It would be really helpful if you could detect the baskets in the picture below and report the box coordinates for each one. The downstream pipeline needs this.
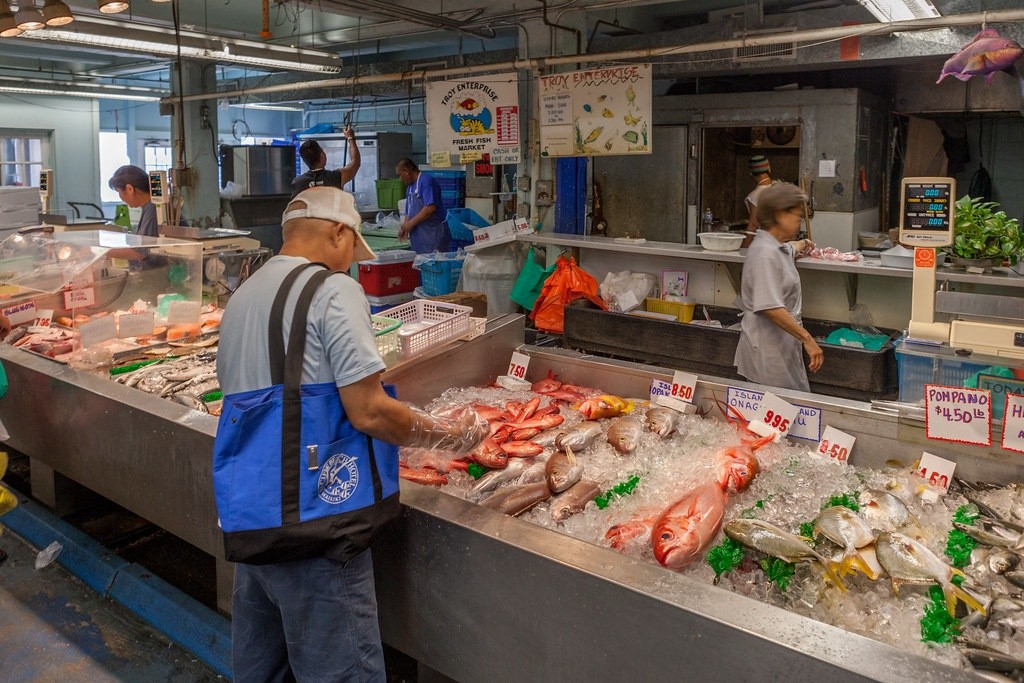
[371,314,403,357]
[646,297,696,323]
[377,298,473,357]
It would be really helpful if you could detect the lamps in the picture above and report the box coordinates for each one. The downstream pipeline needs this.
[43,0,73,26]
[97,0,130,14]
[0,0,24,38]
[19,19,343,76]
[13,0,45,31]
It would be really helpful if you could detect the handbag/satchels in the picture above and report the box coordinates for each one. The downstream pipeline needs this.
[212,262,400,562]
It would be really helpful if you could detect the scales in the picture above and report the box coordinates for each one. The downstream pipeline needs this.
[148,171,261,255]
[898,176,1024,360]
[38,169,108,232]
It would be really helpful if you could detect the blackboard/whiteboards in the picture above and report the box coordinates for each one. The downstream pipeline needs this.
[538,63,652,159]
[426,72,521,163]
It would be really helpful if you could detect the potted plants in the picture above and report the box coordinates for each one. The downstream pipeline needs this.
[945,194,1024,268]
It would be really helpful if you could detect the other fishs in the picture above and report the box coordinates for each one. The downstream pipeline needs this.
[578,394,635,421]
[608,415,642,452]
[859,470,941,538]
[943,472,1024,683]
[714,433,776,495]
[5,298,224,416]
[936,28,1023,86]
[544,450,585,492]
[603,517,653,553]
[548,480,601,525]
[877,530,987,618]
[478,482,553,518]
[393,367,601,487]
[646,406,679,439]
[721,518,859,592]
[556,421,603,452]
[812,505,877,580]
[650,481,729,570]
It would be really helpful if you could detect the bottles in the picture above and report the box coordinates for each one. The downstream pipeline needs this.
[705,208,713,223]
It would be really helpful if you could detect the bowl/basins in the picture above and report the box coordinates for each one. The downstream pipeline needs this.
[697,232,747,251]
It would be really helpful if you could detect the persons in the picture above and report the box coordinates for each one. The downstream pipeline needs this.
[217,187,489,683]
[109,164,189,272]
[741,155,777,248]
[396,158,451,254]
[733,182,824,392]
[291,124,361,200]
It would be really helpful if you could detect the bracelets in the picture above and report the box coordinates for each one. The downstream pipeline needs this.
[348,137,355,142]
[804,239,808,246]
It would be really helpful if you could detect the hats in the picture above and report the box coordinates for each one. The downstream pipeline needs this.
[750,155,771,176]
[279,186,377,261]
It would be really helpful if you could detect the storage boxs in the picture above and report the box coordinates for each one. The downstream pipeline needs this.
[446,207,491,242]
[459,317,486,342]
[421,169,466,218]
[419,253,466,296]
[374,179,405,209]
[350,227,411,280]
[646,295,696,323]
[369,315,401,359]
[359,248,418,297]
[374,300,473,359]
[365,294,415,315]
[895,351,989,404]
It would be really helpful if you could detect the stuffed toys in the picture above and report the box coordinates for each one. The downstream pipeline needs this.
[935,26,1024,85]
